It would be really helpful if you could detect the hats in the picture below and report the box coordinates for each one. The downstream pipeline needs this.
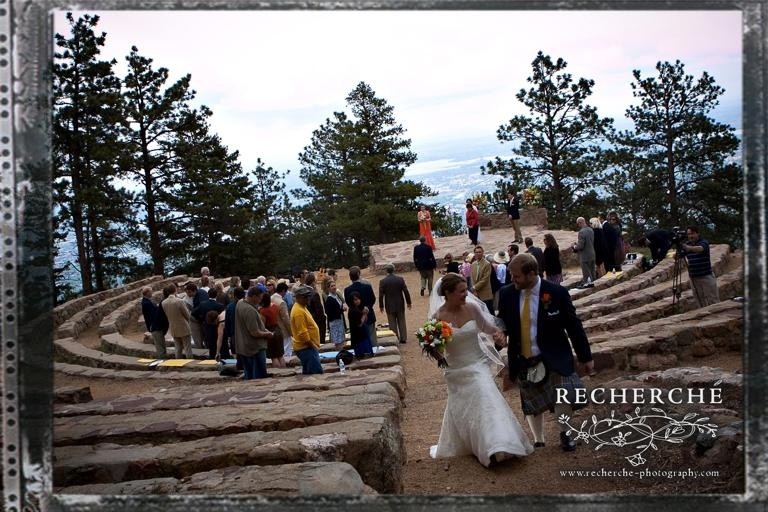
[295,288,311,295]
[493,250,511,264]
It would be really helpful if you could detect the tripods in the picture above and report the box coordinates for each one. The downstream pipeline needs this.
[672,253,703,315]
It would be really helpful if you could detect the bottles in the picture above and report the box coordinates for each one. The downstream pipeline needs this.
[339,359,345,375]
[612,268,614,274]
[586,276,590,284]
[630,255,633,264]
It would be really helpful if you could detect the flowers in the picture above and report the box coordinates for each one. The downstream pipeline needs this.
[410,316,461,370]
[519,183,544,211]
[469,192,490,212]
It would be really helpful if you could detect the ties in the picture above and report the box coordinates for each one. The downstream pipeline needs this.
[519,289,533,360]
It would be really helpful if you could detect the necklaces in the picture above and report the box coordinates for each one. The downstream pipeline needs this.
[453,315,458,322]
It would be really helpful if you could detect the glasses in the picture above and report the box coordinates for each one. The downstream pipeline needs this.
[609,218,616,221]
[265,284,273,287]
[443,256,451,259]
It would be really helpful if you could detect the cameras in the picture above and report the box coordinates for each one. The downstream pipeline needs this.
[571,242,579,254]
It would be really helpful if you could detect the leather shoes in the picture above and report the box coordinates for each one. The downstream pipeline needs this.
[559,429,577,450]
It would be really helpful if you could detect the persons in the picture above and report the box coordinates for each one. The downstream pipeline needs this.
[609,216,625,272]
[588,217,606,277]
[596,211,618,272]
[672,224,720,309]
[139,266,376,382]
[465,198,483,241]
[606,211,625,264]
[417,204,436,252]
[378,263,413,345]
[490,252,598,452]
[412,235,438,298]
[427,270,536,464]
[568,216,597,286]
[507,192,524,244]
[465,203,482,248]
[439,233,564,318]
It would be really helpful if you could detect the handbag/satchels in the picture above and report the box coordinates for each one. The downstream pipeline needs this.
[336,348,354,366]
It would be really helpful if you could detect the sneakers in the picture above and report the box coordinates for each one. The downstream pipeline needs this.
[421,288,424,296]
